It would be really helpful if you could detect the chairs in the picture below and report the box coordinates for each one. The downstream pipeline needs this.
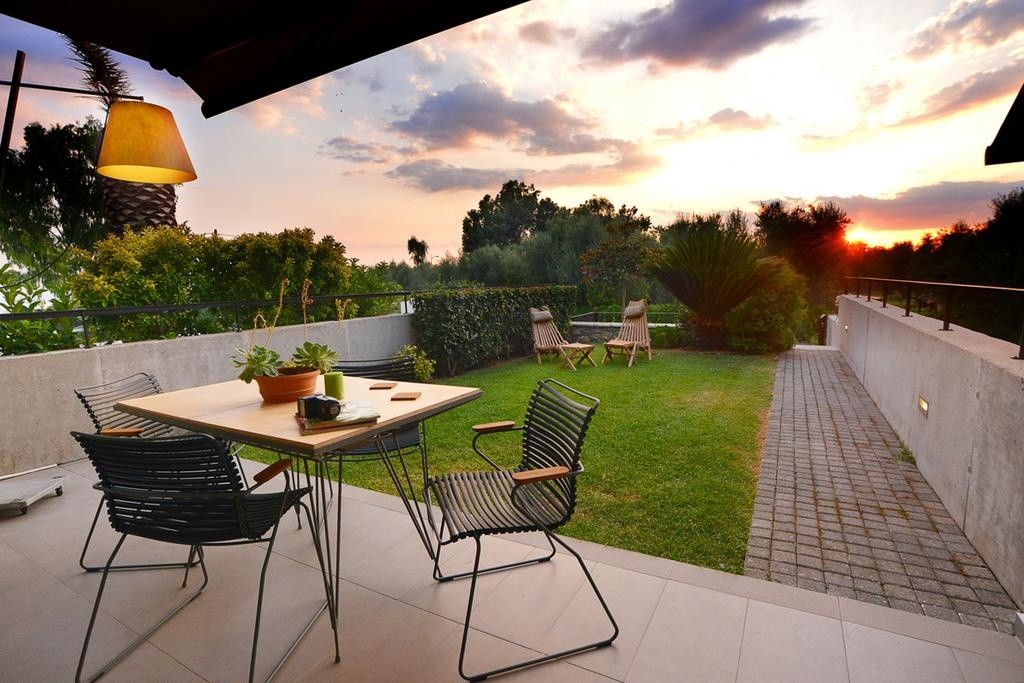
[70,431,333,683]
[529,306,597,371]
[601,299,652,368]
[74,372,251,570]
[315,354,437,533]
[422,378,619,683]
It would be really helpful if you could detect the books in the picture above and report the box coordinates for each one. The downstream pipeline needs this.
[295,399,380,435]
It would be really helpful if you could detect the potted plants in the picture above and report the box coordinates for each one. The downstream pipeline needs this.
[232,342,338,402]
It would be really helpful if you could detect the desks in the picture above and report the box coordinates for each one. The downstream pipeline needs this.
[114,375,482,663]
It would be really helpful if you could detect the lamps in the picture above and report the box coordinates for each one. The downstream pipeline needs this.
[0,80,197,184]
